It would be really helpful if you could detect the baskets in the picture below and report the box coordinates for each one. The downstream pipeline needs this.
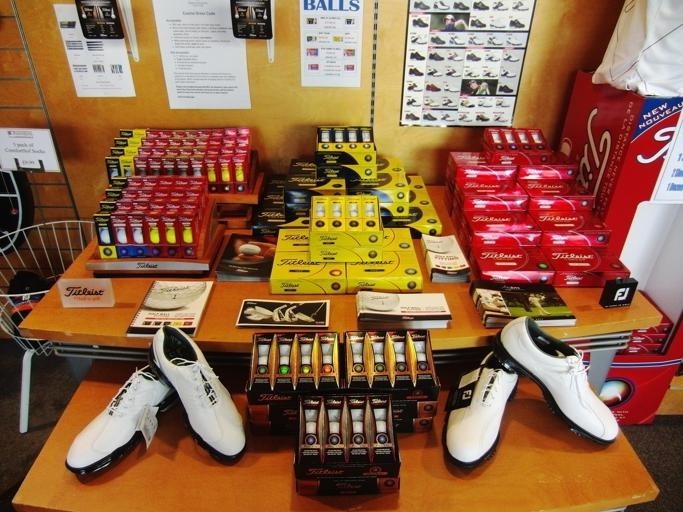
[0,220,92,356]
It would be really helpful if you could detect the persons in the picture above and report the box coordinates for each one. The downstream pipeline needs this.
[442,14,466,31]
[467,80,491,95]
[234,238,277,260]
[516,292,553,318]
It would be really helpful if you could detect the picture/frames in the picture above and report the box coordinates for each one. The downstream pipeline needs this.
[400,0,537,129]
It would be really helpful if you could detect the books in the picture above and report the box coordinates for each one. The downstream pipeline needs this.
[125,278,215,337]
[214,230,278,283]
[354,291,453,330]
[420,230,472,284]
[234,297,331,331]
[467,278,577,329]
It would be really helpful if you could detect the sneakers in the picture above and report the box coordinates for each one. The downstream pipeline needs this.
[65,364,179,476]
[406,0,529,121]
[442,351,520,471]
[492,317,619,445]
[149,325,246,464]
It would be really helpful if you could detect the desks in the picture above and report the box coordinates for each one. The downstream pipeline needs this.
[14,185,663,512]
